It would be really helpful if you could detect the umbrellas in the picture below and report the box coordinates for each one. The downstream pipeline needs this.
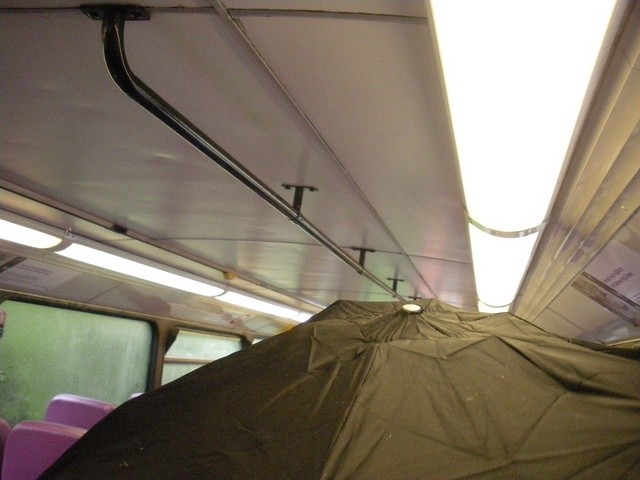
[36,299,640,480]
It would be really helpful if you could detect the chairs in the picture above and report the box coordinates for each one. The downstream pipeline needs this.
[0,418,87,480]
[45,393,117,429]
